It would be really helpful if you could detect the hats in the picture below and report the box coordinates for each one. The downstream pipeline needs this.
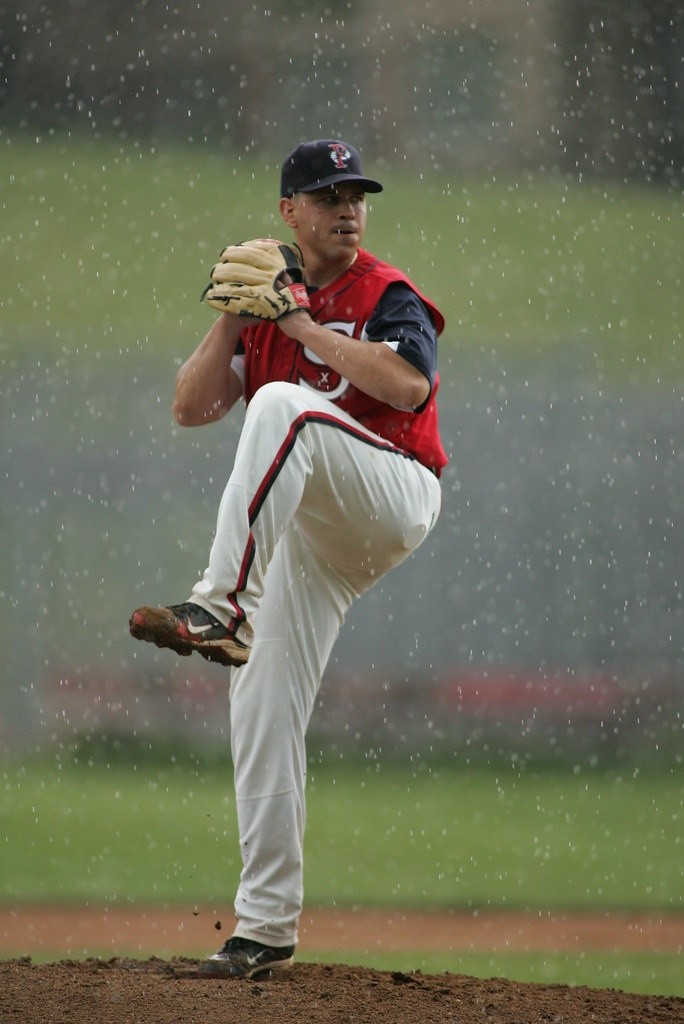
[280,139,383,198]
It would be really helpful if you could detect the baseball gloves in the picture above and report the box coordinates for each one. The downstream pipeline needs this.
[201,235,312,322]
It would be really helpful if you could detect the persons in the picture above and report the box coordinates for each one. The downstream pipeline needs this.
[126,138,448,984]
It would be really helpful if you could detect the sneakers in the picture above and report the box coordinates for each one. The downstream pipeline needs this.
[173,937,295,980]
[129,602,249,667]
